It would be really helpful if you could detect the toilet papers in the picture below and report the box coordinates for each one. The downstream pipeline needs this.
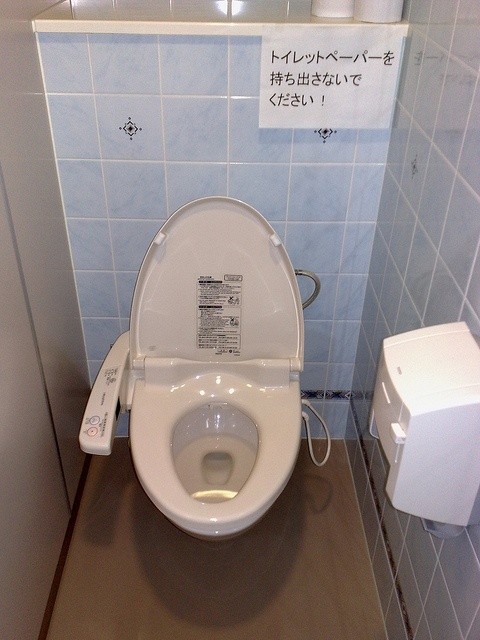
[352,1,404,24]
[311,3,354,19]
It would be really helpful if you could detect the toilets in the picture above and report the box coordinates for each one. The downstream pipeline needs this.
[77,195,305,542]
